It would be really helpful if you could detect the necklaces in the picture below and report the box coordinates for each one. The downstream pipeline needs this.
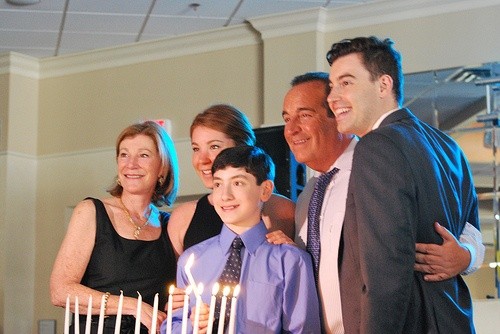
[119,198,154,240]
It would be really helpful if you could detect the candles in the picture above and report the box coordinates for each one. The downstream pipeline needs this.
[182,294,189,334]
[98,295,106,334]
[150,293,159,334]
[207,295,216,334]
[64,296,70,334]
[185,269,203,306]
[166,294,172,334]
[193,295,201,334]
[85,295,92,334]
[135,290,142,334]
[217,296,227,334]
[114,290,124,334]
[228,297,238,334]
[74,296,80,334]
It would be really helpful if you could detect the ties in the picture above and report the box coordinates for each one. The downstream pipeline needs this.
[306,167,340,290]
[212,237,245,334]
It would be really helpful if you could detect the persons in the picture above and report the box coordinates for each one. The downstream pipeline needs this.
[165,104,295,314]
[327,36,480,334]
[160,147,322,334]
[50,121,179,334]
[282,73,485,334]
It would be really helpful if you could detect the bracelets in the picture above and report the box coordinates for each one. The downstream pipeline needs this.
[104,292,110,317]
